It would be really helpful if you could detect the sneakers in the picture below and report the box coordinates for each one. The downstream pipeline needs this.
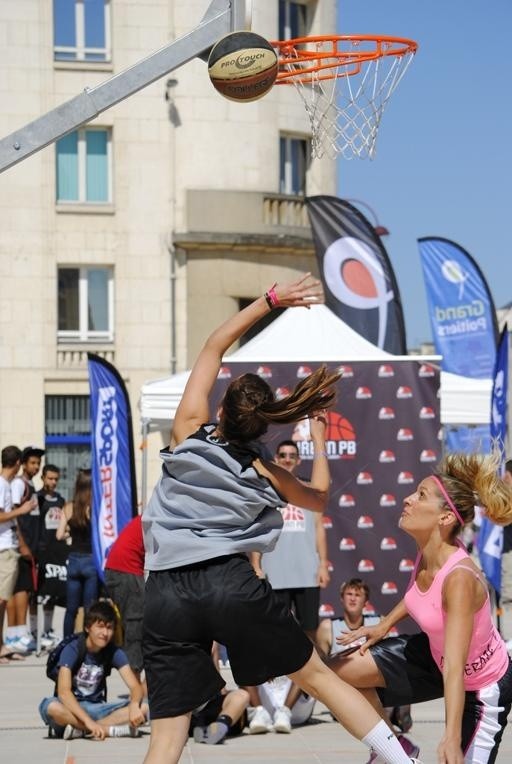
[193,721,228,745]
[114,721,139,738]
[366,735,419,764]
[272,706,291,732]
[4,632,62,652]
[63,724,85,741]
[248,706,271,735]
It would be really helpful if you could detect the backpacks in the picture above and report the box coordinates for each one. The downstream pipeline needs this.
[46,632,118,689]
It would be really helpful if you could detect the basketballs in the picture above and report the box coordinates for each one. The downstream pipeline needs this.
[292,411,355,441]
[208,30,279,103]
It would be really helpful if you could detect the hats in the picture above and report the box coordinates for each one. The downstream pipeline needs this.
[23,446,46,460]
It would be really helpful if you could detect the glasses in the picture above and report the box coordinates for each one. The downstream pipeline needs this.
[278,453,298,458]
